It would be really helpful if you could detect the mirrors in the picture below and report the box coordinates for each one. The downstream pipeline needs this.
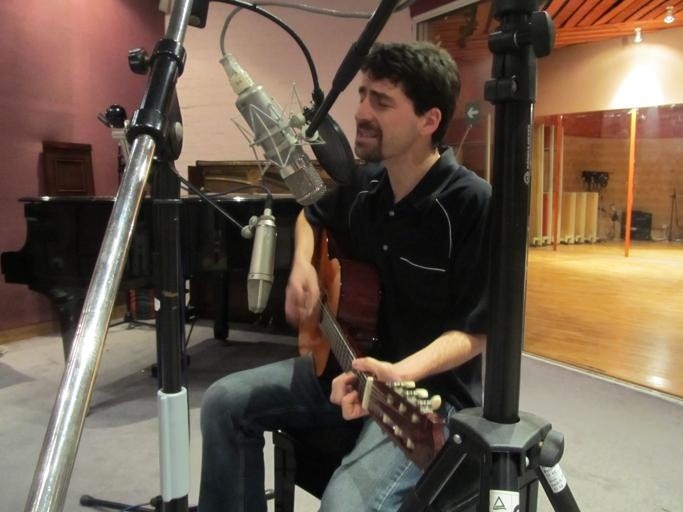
[413,1,683,404]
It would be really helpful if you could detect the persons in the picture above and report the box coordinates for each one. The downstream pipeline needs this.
[199,40,492,512]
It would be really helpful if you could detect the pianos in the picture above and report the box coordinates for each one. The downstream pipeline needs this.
[0,195,303,365]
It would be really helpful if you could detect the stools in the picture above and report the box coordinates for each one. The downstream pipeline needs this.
[273,435,483,512]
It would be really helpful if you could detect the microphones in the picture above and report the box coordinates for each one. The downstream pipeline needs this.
[244,216,278,313]
[235,84,327,206]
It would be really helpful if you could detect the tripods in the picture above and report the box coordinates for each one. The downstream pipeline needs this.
[399,2,579,510]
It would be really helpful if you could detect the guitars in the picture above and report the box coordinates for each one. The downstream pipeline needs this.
[297,225,445,469]
